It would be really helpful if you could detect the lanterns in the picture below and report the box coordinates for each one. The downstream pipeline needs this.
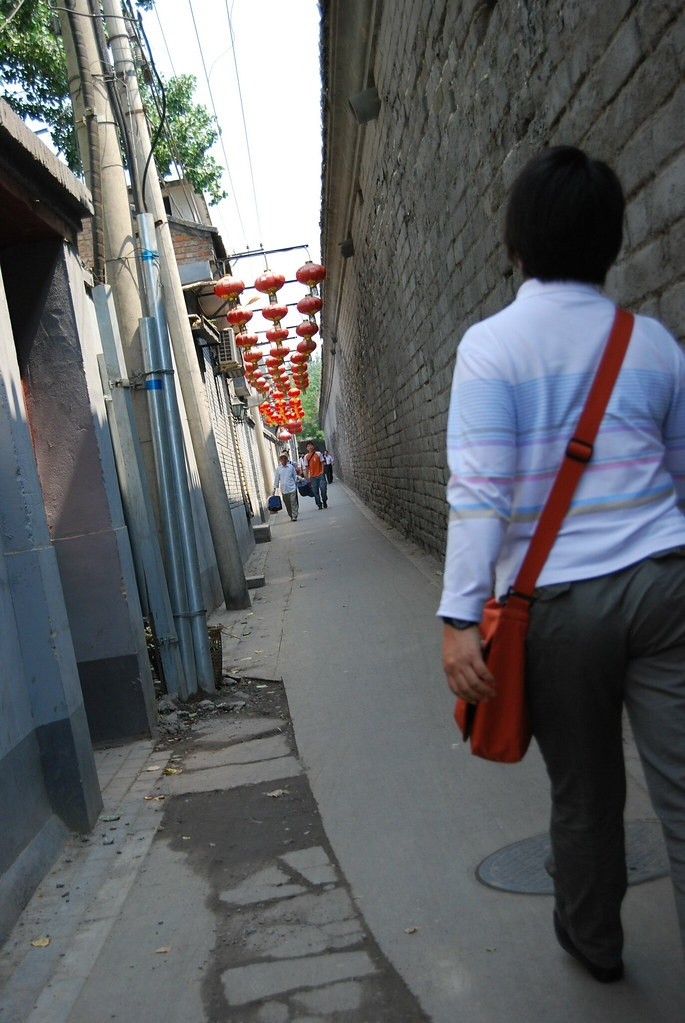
[227,303,253,332]
[270,345,290,360]
[245,352,310,435]
[266,326,289,344]
[214,273,244,301]
[297,293,322,318]
[296,261,326,288]
[279,430,292,442]
[288,388,300,398]
[235,330,258,350]
[262,300,288,325]
[297,339,317,356]
[296,319,318,339]
[243,350,262,365]
[255,269,285,296]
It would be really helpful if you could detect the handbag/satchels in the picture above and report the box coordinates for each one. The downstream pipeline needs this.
[453,597,534,764]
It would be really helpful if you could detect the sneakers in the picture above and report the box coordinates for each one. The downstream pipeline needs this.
[291,517,297,521]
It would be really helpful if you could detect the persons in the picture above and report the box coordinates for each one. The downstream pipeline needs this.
[432,146,685,983]
[278,450,299,508]
[272,452,298,521]
[304,440,328,510]
[298,450,336,484]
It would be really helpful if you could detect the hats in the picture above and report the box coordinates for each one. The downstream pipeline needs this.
[280,453,288,458]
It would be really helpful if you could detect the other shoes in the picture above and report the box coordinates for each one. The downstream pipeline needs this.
[318,502,323,510]
[323,502,327,509]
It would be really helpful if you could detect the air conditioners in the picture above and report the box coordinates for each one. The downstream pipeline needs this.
[217,327,239,369]
[233,376,252,398]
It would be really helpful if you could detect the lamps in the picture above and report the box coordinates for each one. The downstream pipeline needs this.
[230,395,245,423]
[236,406,249,424]
